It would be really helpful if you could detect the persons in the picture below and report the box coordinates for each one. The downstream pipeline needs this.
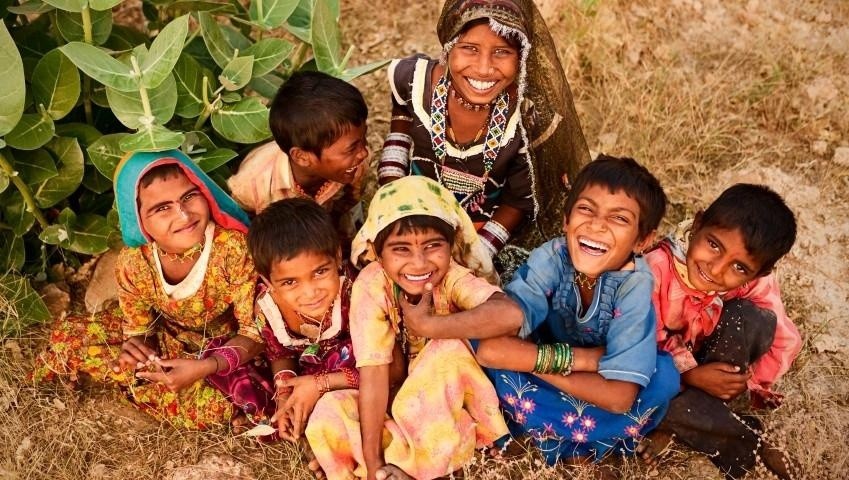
[200,197,362,448]
[222,70,374,263]
[376,0,552,257]
[643,182,808,480]
[470,153,686,480]
[33,147,272,428]
[305,174,525,480]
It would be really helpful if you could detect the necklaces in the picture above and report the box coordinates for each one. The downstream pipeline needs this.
[448,81,498,112]
[152,236,207,264]
[294,302,335,358]
[575,269,597,290]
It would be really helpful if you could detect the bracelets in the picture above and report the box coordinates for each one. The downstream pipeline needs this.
[273,368,298,401]
[314,367,330,396]
[210,346,240,377]
[209,355,219,375]
[532,342,575,379]
